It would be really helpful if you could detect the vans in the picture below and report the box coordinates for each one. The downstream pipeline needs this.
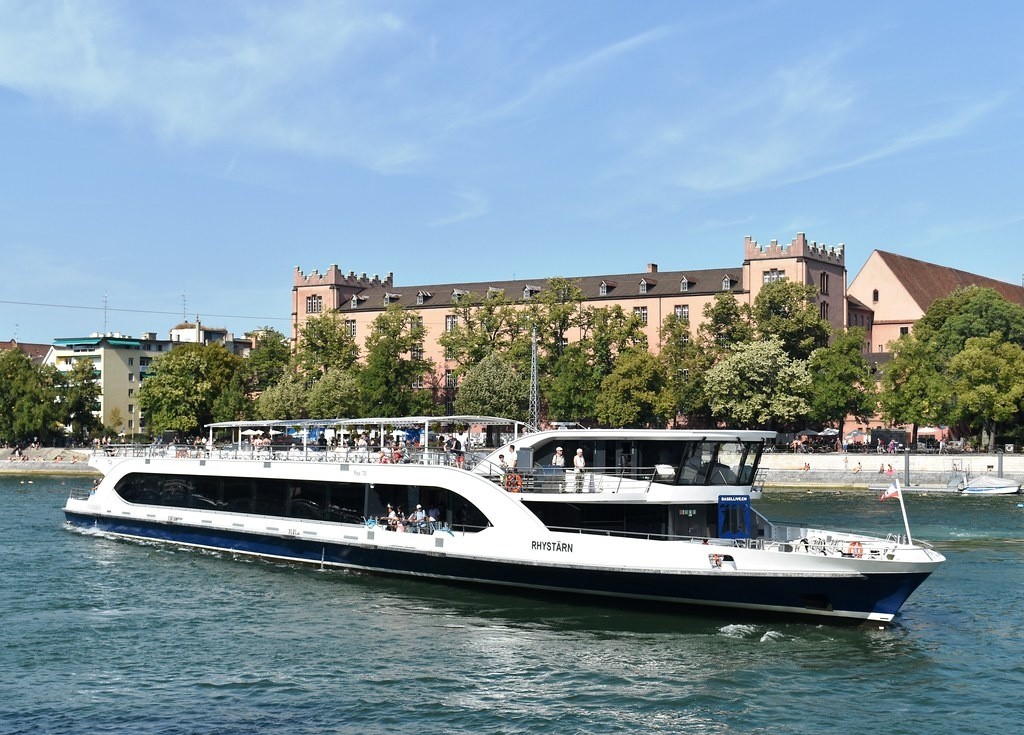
[947,441,964,453]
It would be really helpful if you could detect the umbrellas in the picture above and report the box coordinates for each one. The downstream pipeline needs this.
[849,430,862,435]
[799,428,819,435]
[817,428,840,435]
[918,427,938,448]
[241,428,309,438]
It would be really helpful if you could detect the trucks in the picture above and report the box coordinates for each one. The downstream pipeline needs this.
[722,442,746,453]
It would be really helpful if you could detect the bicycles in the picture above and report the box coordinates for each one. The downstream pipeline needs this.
[988,445,1003,456]
[817,444,833,453]
[798,444,814,454]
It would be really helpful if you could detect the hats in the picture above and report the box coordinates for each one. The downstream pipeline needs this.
[577,448,582,453]
[417,504,421,509]
[556,447,563,450]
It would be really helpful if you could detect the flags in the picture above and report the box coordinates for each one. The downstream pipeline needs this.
[879,480,900,503]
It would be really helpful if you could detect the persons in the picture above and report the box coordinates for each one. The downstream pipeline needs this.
[498,454,508,486]
[789,435,898,454]
[844,456,848,470]
[853,462,862,474]
[206,438,213,457]
[254,427,469,464]
[878,463,884,473]
[802,463,810,471]
[508,445,518,474]
[887,464,892,472]
[166,442,176,458]
[573,448,586,493]
[522,427,528,437]
[381,504,440,534]
[552,447,566,492]
[539,422,551,432]
[102,436,114,456]
[194,437,203,457]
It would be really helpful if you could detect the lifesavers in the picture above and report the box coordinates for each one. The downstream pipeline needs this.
[380,453,385,462]
[177,450,190,458]
[853,467,859,473]
[506,473,522,491]
[848,542,863,558]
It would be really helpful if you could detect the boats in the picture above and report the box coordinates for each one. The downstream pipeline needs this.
[957,473,1020,496]
[62,417,947,628]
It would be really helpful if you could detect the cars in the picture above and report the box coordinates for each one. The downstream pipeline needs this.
[750,444,771,453]
